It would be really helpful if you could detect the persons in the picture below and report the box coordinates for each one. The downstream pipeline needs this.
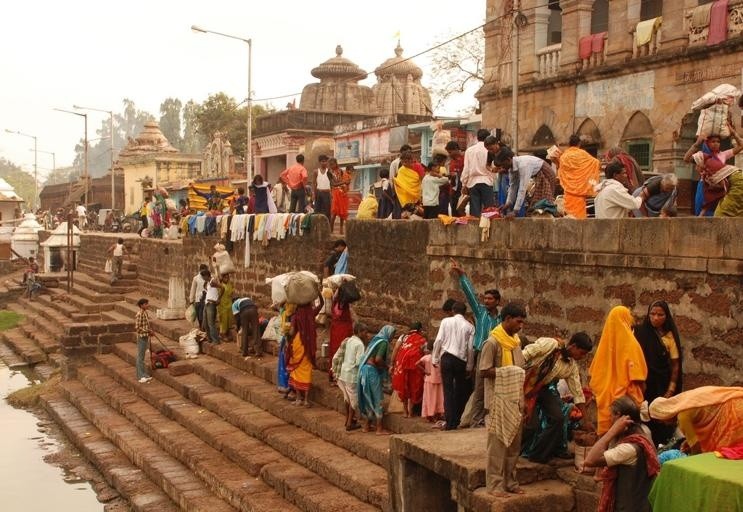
[190,239,502,435]
[24,256,42,302]
[108,238,129,283]
[135,298,154,384]
[30,154,354,239]
[355,120,743,220]
[479,300,743,512]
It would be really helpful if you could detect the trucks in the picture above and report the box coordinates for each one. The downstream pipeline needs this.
[95,209,124,231]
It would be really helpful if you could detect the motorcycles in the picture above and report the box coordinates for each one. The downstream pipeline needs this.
[106,215,131,233]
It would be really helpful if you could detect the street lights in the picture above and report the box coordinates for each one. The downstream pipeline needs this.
[72,105,116,208]
[4,128,41,211]
[25,146,56,188]
[189,24,252,199]
[50,106,89,207]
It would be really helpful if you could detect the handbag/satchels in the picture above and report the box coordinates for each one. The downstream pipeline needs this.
[694,95,730,141]
[148,333,176,371]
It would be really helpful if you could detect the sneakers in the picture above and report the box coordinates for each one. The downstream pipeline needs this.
[136,376,152,384]
[276,385,292,395]
[344,418,361,431]
[290,399,313,408]
